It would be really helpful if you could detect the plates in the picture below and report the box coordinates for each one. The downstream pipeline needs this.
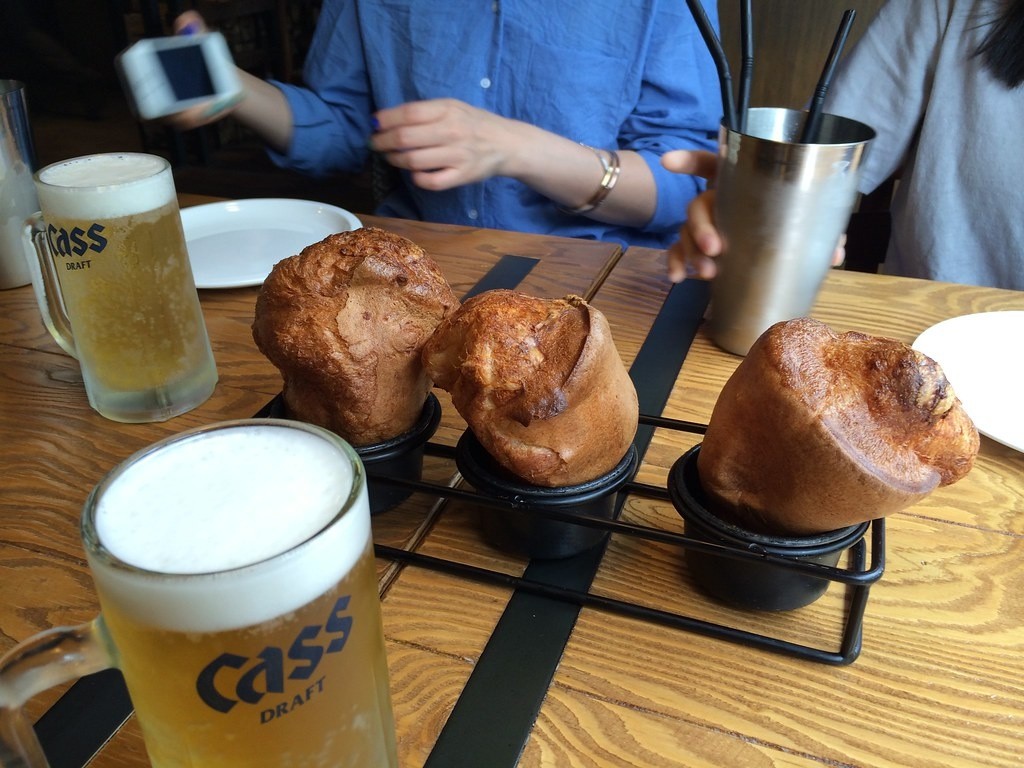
[179,196,364,288]
[911,311,1024,454]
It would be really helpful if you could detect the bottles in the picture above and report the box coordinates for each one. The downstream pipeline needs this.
[0,98,45,290]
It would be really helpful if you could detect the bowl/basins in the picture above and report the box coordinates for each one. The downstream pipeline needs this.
[668,441,871,612]
[453,426,640,562]
[352,391,442,515]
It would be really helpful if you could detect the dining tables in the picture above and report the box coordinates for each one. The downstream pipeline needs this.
[1,190,1023,768]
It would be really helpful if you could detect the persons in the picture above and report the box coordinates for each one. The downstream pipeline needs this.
[660,0,1024,291]
[169,0,725,250]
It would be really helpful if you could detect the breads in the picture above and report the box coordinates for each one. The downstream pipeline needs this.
[419,288,639,487]
[698,316,979,536]
[253,228,459,449]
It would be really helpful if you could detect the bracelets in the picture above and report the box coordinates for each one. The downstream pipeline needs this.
[558,143,619,214]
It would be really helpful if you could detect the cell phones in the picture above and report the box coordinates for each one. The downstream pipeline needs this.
[124,31,237,121]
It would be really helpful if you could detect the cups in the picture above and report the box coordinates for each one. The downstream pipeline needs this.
[0,418,396,768]
[0,79,34,172]
[22,152,219,423]
[710,106,879,360]
